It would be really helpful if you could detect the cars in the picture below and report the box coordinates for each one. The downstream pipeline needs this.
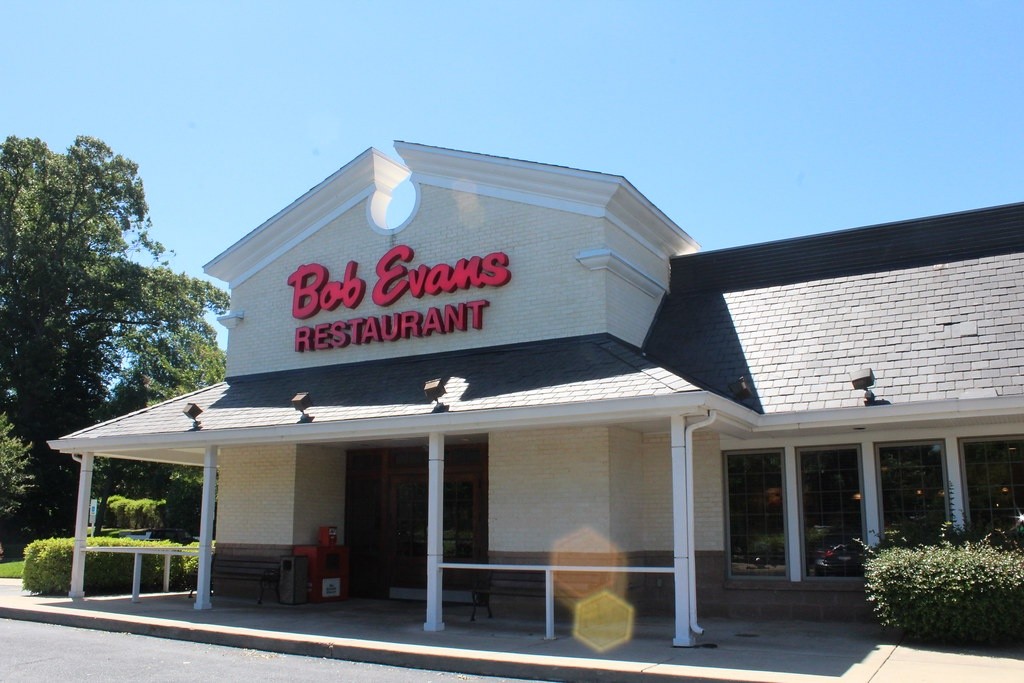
[148,528,192,544]
[810,537,865,578]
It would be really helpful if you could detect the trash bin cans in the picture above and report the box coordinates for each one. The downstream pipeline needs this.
[278,555,309,604]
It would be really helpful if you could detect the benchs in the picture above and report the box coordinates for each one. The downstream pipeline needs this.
[184,548,285,607]
[469,572,571,621]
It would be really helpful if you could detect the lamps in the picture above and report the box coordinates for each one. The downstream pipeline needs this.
[850,368,875,403]
[183,403,203,427]
[291,392,310,420]
[424,378,446,410]
